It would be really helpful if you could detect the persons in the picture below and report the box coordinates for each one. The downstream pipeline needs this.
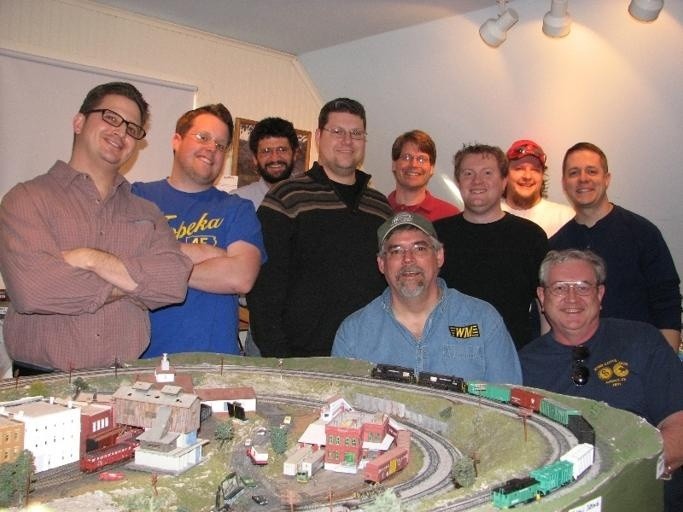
[532,141,678,351]
[499,141,576,238]
[384,128,462,226]
[1,83,187,372]
[512,249,681,472]
[223,116,299,353]
[247,100,398,356]
[124,102,268,355]
[425,143,547,349]
[327,212,523,386]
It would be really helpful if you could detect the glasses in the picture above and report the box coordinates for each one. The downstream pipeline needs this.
[183,131,231,154]
[85,108,147,141]
[255,147,291,156]
[512,146,546,162]
[383,241,433,258]
[321,127,368,140]
[542,280,597,297]
[396,153,429,164]
[569,345,590,386]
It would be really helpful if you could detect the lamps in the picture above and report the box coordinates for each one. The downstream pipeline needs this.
[475,0,664,49]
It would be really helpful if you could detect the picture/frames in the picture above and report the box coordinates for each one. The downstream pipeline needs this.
[229,117,312,190]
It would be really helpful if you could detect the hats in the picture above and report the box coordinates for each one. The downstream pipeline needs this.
[506,139,546,168]
[376,210,438,249]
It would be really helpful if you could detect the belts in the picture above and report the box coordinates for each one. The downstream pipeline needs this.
[11,361,55,379]
